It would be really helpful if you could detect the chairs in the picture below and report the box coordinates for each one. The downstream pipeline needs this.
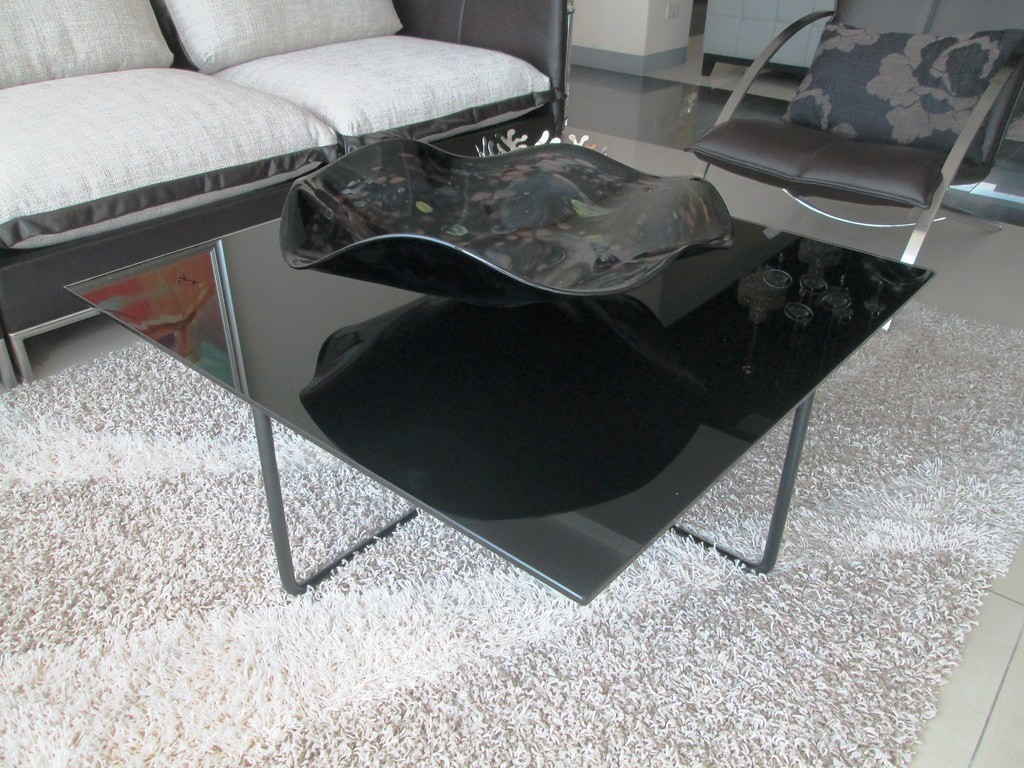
[682,0,1024,274]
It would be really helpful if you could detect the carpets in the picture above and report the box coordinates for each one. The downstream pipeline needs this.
[0,300,1024,768]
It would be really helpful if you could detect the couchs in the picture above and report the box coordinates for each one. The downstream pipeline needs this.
[702,0,836,75]
[0,0,573,391]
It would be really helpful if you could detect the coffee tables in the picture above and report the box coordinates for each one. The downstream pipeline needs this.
[63,151,935,604]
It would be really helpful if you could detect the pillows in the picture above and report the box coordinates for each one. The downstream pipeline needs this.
[789,22,1024,167]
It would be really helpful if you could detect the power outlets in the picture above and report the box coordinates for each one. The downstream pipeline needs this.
[666,2,679,18]
